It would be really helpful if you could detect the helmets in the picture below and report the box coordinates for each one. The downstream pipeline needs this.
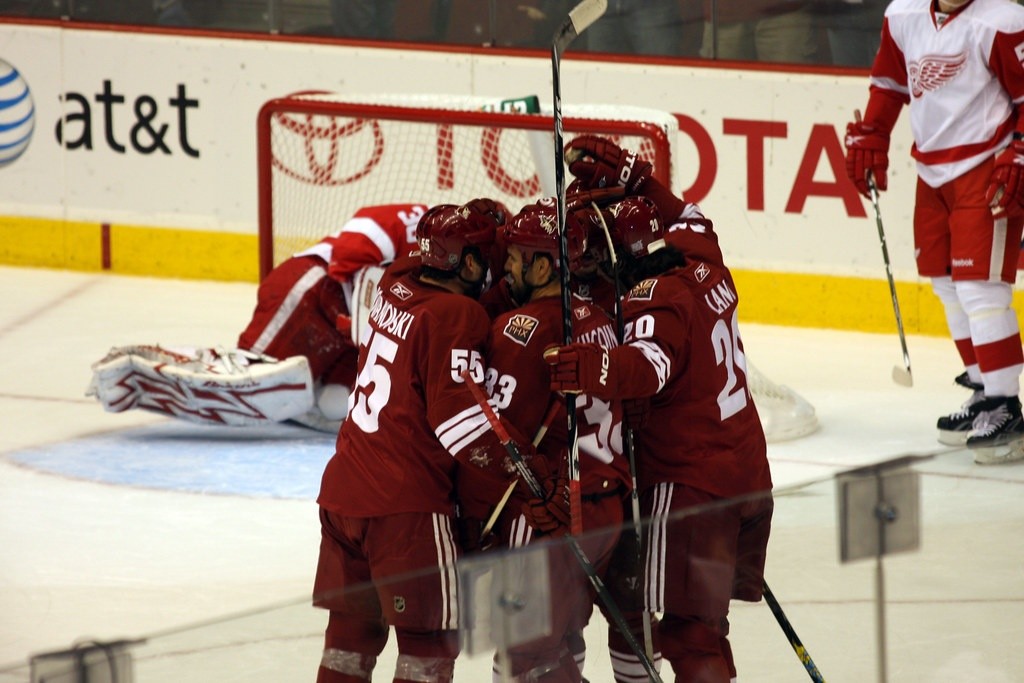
[415,194,667,290]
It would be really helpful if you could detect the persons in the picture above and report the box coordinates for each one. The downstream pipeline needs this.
[461,178,661,683]
[311,204,589,683]
[845,0,1024,466]
[461,204,632,683]
[544,135,773,683]
[0,0,890,69]
[91,204,428,425]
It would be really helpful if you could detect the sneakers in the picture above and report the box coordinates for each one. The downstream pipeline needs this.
[936,370,1024,465]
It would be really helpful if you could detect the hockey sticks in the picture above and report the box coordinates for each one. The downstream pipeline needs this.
[463,357,666,683]
[757,581,821,683]
[470,394,566,555]
[552,2,609,538]
[864,169,912,389]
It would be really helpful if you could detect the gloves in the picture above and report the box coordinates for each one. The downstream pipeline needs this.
[542,342,619,405]
[984,140,1024,221]
[844,121,891,202]
[563,135,653,194]
[517,476,571,546]
